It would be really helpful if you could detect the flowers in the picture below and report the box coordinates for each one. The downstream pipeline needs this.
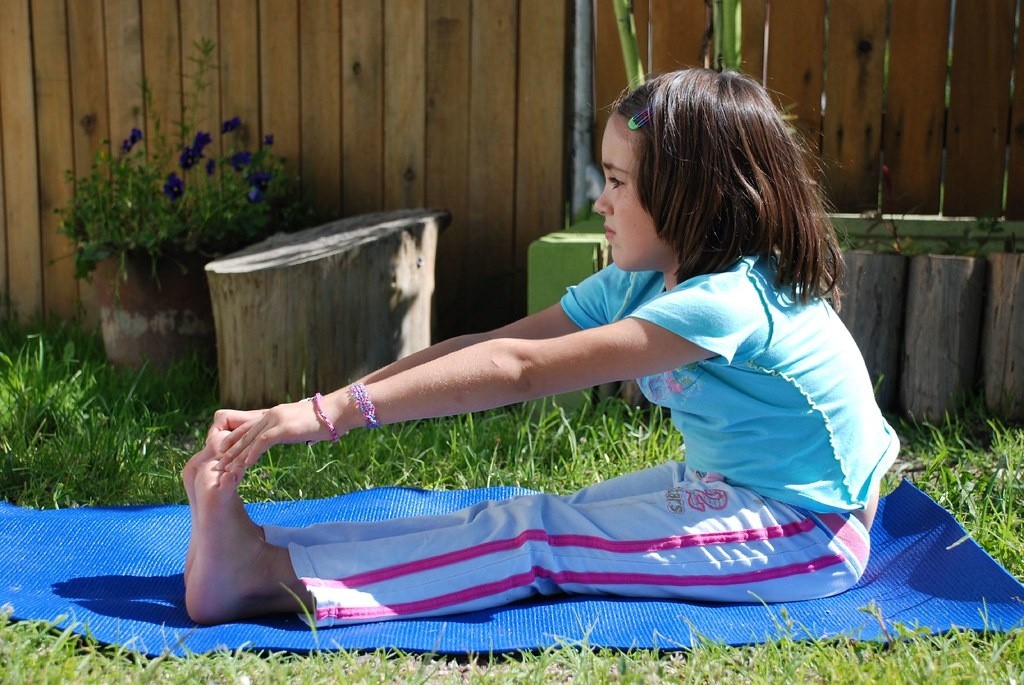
[42,33,317,302]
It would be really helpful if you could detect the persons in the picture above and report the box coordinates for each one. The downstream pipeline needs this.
[182,65,902,631]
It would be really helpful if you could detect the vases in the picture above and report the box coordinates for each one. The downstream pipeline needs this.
[89,254,217,365]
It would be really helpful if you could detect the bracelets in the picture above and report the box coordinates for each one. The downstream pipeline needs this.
[308,392,339,447]
[348,382,382,429]
